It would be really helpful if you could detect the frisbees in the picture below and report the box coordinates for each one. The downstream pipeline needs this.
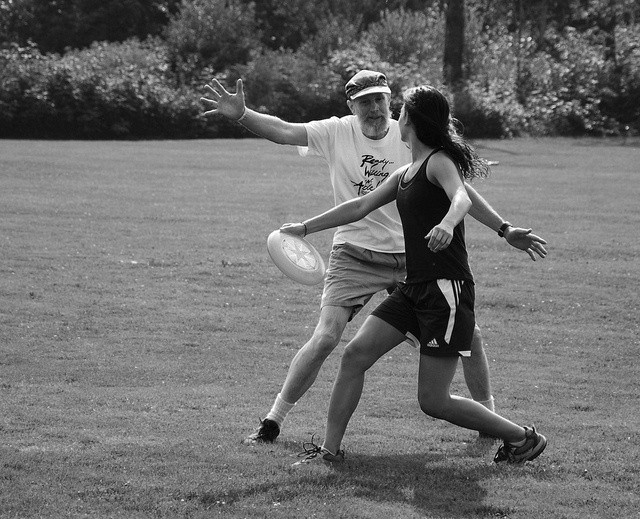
[266,229,327,285]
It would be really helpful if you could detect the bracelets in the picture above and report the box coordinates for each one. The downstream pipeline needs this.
[236,106,247,123]
[300,222,308,237]
[498,221,513,237]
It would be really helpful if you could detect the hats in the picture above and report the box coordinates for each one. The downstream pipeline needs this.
[345,70,392,99]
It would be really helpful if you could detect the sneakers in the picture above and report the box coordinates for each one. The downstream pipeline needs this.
[292,445,345,465]
[491,425,547,465]
[244,418,280,444]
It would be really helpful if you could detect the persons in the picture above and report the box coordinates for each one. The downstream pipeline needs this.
[200,69,549,446]
[280,85,548,469]
